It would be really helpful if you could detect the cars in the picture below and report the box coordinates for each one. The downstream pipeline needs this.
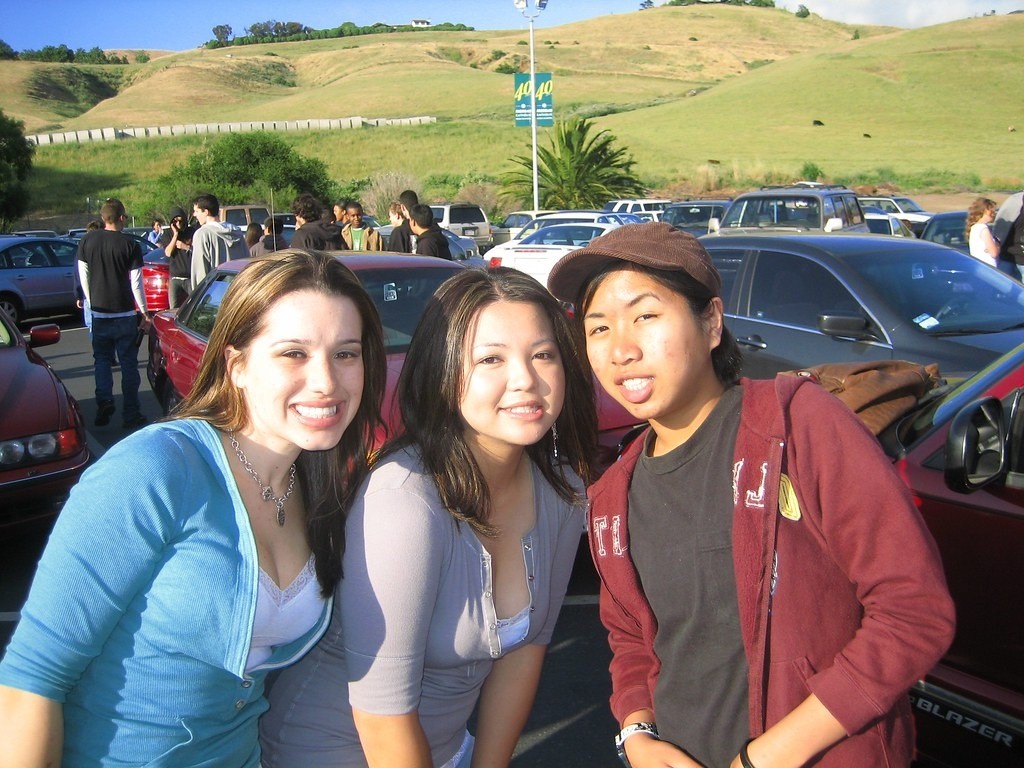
[145,248,650,598]
[691,230,1024,400]
[874,336,1024,768]
[0,304,94,544]
[1,180,1023,322]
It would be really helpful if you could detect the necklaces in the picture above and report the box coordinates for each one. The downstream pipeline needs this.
[227,430,297,527]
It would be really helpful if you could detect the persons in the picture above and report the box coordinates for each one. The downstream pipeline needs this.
[190,193,251,291]
[249,216,289,258]
[290,194,350,251]
[163,206,197,309]
[548,222,955,768]
[340,202,386,250]
[0,248,390,768]
[334,198,350,228]
[320,210,336,224]
[147,219,170,251]
[259,263,603,768]
[244,223,263,248]
[388,200,407,252]
[73,220,138,367]
[409,204,453,261]
[392,189,441,253]
[964,197,1000,269]
[77,198,152,429]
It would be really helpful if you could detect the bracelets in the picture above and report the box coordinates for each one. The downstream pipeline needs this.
[739,737,755,768]
[185,245,190,254]
[615,722,659,768]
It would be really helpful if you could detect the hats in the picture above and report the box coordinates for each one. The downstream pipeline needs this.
[549,222,722,303]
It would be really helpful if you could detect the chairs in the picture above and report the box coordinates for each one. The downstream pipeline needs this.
[766,204,787,224]
[943,231,966,245]
[29,252,49,268]
[377,269,428,336]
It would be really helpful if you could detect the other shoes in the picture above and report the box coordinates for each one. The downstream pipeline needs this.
[95,400,116,427]
[123,414,147,430]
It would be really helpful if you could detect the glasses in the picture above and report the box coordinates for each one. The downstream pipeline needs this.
[173,220,184,224]
[123,215,128,219]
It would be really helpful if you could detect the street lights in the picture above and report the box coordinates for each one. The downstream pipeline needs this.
[513,0,549,213]
